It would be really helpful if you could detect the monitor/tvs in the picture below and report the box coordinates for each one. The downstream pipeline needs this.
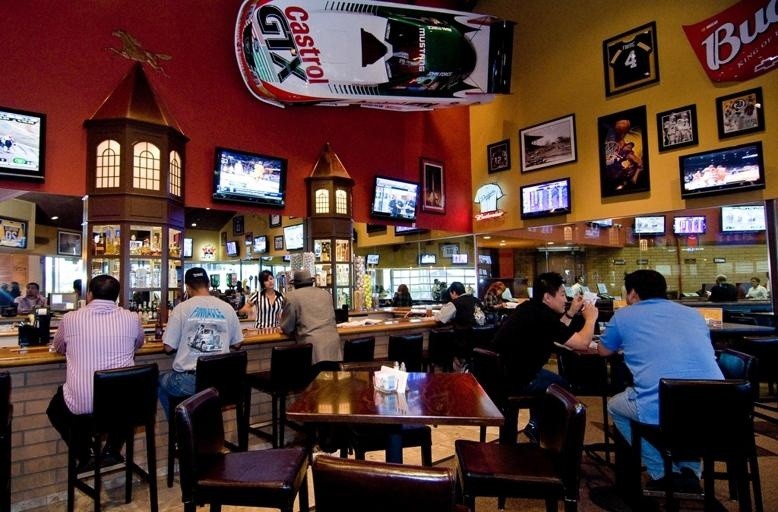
[584,203,770,239]
[173,224,309,264]
[597,282,608,296]
[369,175,421,220]
[678,139,767,200]
[210,145,289,209]
[350,224,469,268]
[0,106,48,184]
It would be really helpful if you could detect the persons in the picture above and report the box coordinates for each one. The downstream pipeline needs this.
[73,279,83,298]
[235,278,242,293]
[477,269,598,441]
[484,280,508,319]
[707,273,738,303]
[15,282,49,316]
[280,269,345,380]
[0,281,19,308]
[235,270,284,333]
[568,274,587,297]
[594,268,727,491]
[723,96,758,133]
[743,276,769,300]
[662,111,693,145]
[45,273,146,473]
[434,280,485,364]
[156,264,244,428]
[606,134,643,191]
[390,283,413,307]
[687,164,738,188]
[389,195,415,217]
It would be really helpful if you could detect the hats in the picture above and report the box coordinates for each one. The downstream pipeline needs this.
[184,267,209,284]
[288,269,317,286]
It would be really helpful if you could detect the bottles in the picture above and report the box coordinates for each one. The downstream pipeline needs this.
[313,242,350,310]
[154,317,163,339]
[91,226,181,256]
[289,252,316,282]
[168,302,173,317]
[128,300,157,319]
[113,260,177,287]
[353,255,380,311]
[392,361,409,392]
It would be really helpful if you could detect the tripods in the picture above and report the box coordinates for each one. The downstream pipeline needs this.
[519,177,572,219]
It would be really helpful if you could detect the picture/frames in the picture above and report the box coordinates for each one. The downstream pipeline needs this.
[215,209,283,256]
[601,19,663,99]
[55,229,82,258]
[418,156,448,216]
[655,103,700,154]
[486,138,512,176]
[713,85,765,140]
[518,111,580,176]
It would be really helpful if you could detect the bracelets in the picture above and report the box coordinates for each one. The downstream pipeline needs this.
[565,311,573,319]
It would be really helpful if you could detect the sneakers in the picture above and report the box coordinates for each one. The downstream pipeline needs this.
[524,420,539,445]
[75,451,112,480]
[92,451,127,472]
[646,470,685,494]
[680,466,704,494]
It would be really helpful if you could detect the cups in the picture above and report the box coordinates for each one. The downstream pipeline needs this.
[426,307,432,317]
[36,315,51,343]
[705,318,721,328]
[19,325,38,345]
[371,373,397,394]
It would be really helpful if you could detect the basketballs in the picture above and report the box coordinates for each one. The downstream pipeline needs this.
[613,119,630,136]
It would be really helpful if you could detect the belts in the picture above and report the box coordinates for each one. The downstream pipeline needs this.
[178,368,198,375]
